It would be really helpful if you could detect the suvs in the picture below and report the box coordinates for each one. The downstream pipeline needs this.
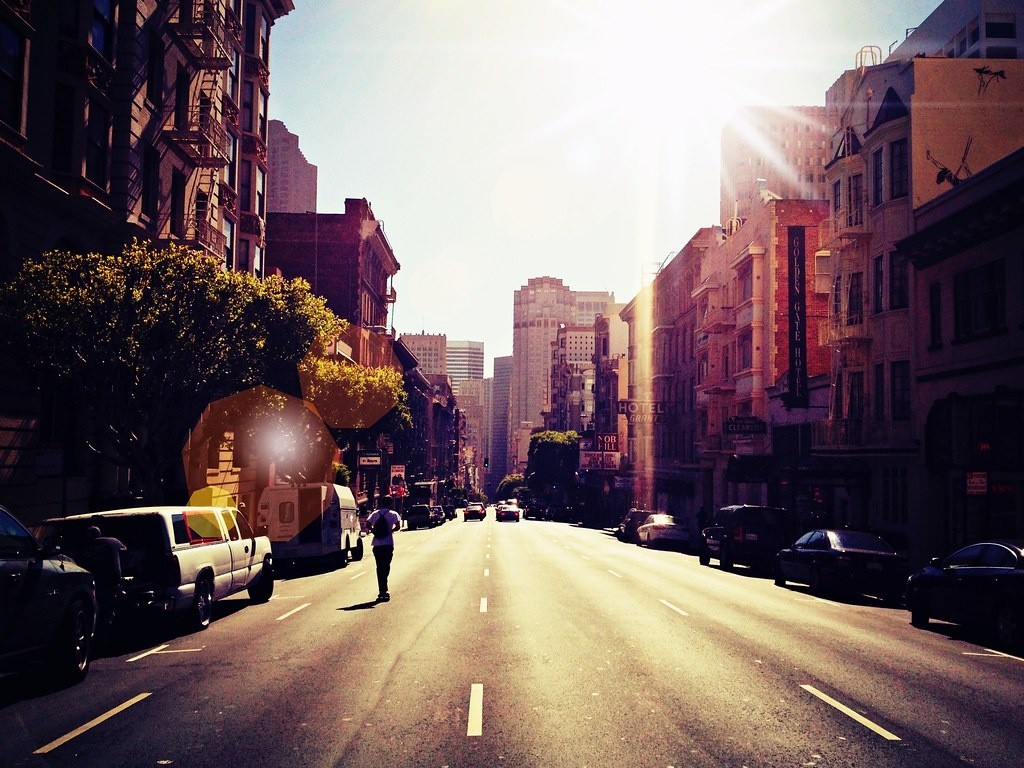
[618,508,656,542]
[701,504,788,575]
[0,504,98,690]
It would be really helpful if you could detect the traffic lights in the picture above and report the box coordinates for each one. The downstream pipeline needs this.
[483,457,490,467]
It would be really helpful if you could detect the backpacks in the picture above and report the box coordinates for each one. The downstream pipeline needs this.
[373,510,390,540]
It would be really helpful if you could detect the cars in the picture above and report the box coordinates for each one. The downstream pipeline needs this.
[905,540,1024,637]
[774,529,906,604]
[406,502,545,530]
[636,513,690,549]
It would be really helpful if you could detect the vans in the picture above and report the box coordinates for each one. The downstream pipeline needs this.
[35,504,274,638]
[257,481,364,571]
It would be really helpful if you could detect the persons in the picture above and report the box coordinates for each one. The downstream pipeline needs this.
[364,495,401,603]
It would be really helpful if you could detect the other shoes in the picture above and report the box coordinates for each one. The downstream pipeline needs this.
[378,592,390,603]
